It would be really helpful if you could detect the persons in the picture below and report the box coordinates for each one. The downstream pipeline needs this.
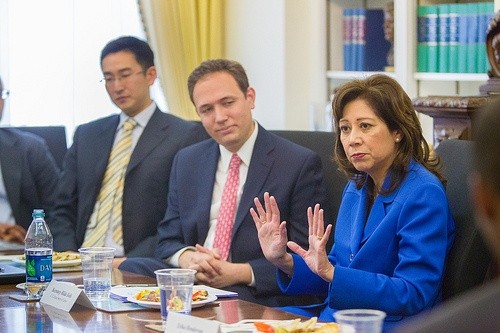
[51,35,212,275]
[398,103,500,333]
[0,77,60,250]
[250,75,456,333]
[155,59,327,307]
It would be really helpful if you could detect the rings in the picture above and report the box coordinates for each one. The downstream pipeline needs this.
[317,235,322,240]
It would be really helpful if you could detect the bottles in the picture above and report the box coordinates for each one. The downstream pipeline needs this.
[24,209,54,296]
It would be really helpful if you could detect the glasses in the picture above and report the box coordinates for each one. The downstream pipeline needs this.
[101,70,144,84]
[0,88,10,100]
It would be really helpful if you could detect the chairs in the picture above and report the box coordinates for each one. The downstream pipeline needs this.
[9,125,68,173]
[433,138,496,304]
[265,129,349,239]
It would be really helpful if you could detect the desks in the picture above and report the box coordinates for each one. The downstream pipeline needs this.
[0,266,331,333]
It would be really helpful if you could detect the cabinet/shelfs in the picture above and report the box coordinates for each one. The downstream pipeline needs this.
[317,0,500,131]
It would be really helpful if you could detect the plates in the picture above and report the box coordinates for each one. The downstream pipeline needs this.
[126,291,217,310]
[12,255,82,268]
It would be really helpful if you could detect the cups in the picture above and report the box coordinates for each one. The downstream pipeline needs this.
[153,269,197,330]
[78,247,116,302]
[333,309,387,333]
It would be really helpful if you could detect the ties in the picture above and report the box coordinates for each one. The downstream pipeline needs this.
[211,152,243,262]
[82,118,139,249]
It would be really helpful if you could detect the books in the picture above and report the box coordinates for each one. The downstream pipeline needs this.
[416,3,494,76]
[343,8,389,73]
